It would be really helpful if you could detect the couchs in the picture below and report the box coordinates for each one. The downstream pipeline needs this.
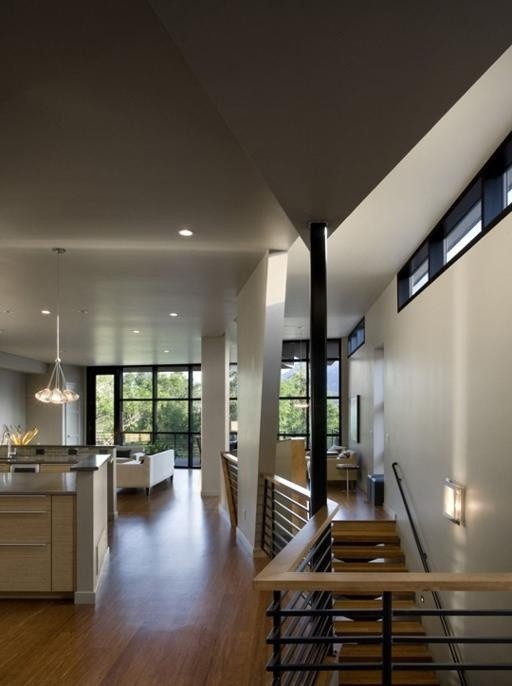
[116,449,175,498]
[306,446,356,491]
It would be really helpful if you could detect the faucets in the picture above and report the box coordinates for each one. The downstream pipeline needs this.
[1,430,17,458]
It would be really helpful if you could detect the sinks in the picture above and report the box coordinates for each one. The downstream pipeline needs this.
[0,457,18,463]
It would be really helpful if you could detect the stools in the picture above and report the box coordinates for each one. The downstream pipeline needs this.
[367,473,383,504]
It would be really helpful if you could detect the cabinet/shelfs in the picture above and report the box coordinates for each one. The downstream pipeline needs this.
[1,493,76,594]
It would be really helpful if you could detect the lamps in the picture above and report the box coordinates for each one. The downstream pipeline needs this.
[34,247,80,403]
[439,477,465,528]
[230,421,238,440]
[295,327,309,408]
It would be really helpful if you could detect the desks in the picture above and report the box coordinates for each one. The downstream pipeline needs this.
[337,464,360,494]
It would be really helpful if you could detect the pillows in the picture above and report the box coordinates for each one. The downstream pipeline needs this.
[327,448,350,459]
